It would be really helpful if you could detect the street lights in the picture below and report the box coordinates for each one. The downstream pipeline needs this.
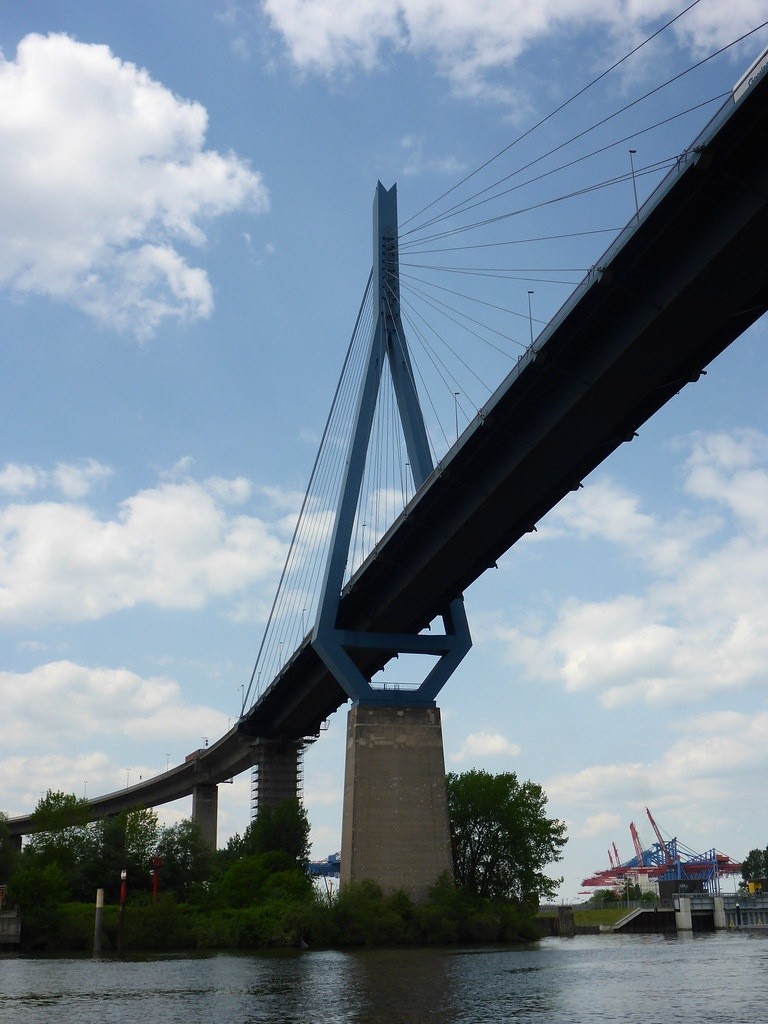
[405,462,412,507]
[629,148,638,212]
[302,607,307,641]
[258,671,262,701]
[453,390,461,443]
[165,753,171,774]
[125,768,132,788]
[279,640,283,672]
[526,289,536,346]
[83,780,89,799]
[360,522,368,563]
